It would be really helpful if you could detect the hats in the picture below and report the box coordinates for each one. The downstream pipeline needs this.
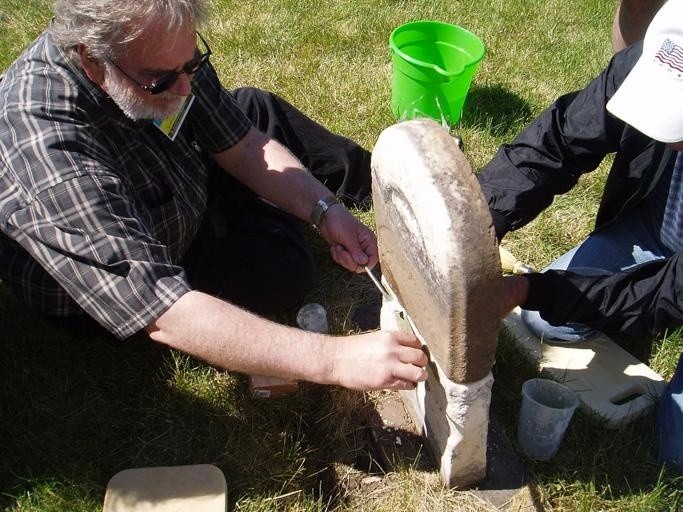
[605,1,683,145]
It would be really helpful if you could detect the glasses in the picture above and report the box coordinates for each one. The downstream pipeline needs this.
[105,29,212,95]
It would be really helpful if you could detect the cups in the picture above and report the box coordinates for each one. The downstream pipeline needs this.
[518,377,579,464]
[296,302,331,337]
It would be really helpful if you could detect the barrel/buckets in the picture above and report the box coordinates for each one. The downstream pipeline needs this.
[388,20,485,128]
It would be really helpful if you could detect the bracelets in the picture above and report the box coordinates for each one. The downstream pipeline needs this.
[310,194,342,236]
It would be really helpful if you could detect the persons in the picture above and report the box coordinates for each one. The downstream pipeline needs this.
[1,2,430,393]
[474,2,683,473]
